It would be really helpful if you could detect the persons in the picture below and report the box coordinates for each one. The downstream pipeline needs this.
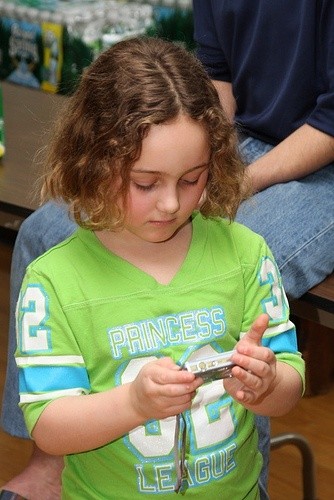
[0,0,333,500]
[0,0,334,500]
[12,31,307,499]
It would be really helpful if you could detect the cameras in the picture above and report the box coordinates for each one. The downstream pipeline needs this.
[182,350,248,383]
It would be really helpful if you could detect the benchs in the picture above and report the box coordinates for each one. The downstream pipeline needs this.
[0,79,334,315]
